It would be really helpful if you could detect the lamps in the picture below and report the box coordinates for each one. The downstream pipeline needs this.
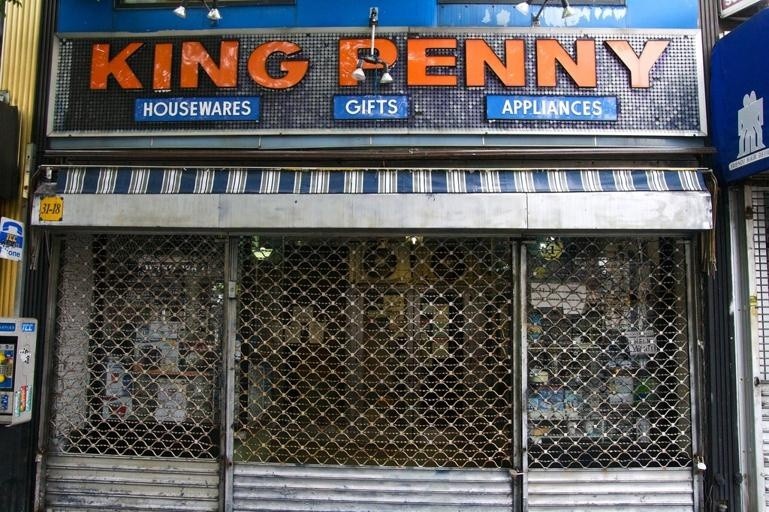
[514,0,575,26]
[350,8,394,85]
[173,1,222,25]
